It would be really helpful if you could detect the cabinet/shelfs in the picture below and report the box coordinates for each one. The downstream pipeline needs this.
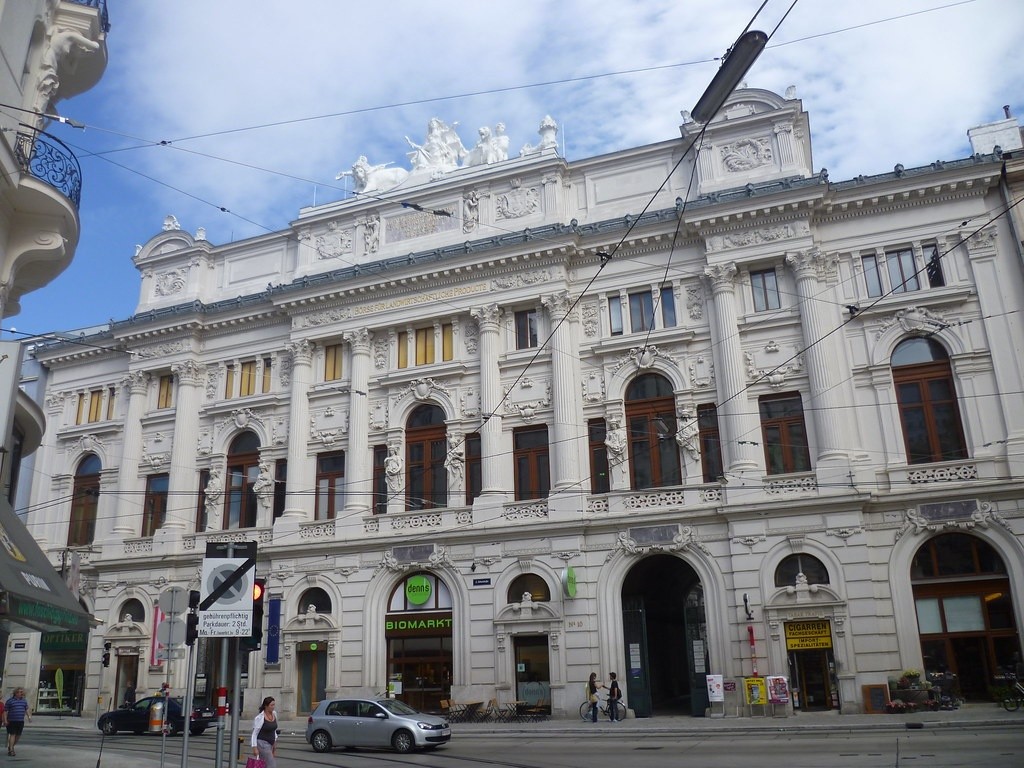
[36,688,72,712]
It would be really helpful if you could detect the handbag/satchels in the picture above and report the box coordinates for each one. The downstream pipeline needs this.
[246,754,267,768]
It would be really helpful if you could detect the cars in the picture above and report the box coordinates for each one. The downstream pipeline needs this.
[304,697,452,755]
[97,696,217,738]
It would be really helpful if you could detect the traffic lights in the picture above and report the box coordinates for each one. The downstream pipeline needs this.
[186,613,199,646]
[101,652,111,667]
[240,577,267,651]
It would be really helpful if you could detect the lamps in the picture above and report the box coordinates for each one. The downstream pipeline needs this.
[691,30,768,124]
[651,418,671,434]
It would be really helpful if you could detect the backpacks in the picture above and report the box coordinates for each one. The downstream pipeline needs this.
[617,687,622,700]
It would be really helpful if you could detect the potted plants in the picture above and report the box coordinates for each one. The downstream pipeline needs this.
[888,675,898,689]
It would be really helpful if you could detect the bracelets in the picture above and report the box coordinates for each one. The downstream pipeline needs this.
[28,716,31,719]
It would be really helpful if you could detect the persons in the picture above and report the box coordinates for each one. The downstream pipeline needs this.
[675,409,700,463]
[202,468,223,516]
[250,697,278,768]
[252,462,272,509]
[602,672,621,721]
[383,444,403,495]
[604,417,628,483]
[584,672,603,723]
[122,680,136,709]
[443,436,466,492]
[334,118,510,197]
[0,686,32,757]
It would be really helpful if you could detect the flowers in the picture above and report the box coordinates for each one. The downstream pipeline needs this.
[898,677,911,687]
[922,698,942,707]
[886,698,919,709]
[904,669,920,678]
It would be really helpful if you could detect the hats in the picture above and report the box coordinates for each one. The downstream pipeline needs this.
[610,672,616,679]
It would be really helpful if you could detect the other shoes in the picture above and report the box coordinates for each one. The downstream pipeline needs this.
[8,750,16,756]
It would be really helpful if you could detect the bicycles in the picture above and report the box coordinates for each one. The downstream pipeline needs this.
[579,694,626,722]
[1001,670,1024,712]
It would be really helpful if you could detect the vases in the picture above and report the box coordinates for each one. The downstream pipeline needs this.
[911,677,920,685]
[925,705,939,711]
[901,686,910,689]
[889,707,915,713]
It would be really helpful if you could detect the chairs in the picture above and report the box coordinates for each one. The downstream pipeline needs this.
[440,698,464,723]
[525,698,551,724]
[476,698,508,724]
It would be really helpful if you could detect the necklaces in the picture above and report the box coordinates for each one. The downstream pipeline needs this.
[264,712,273,722]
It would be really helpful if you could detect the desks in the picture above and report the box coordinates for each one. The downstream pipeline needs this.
[503,701,528,723]
[455,701,484,723]
